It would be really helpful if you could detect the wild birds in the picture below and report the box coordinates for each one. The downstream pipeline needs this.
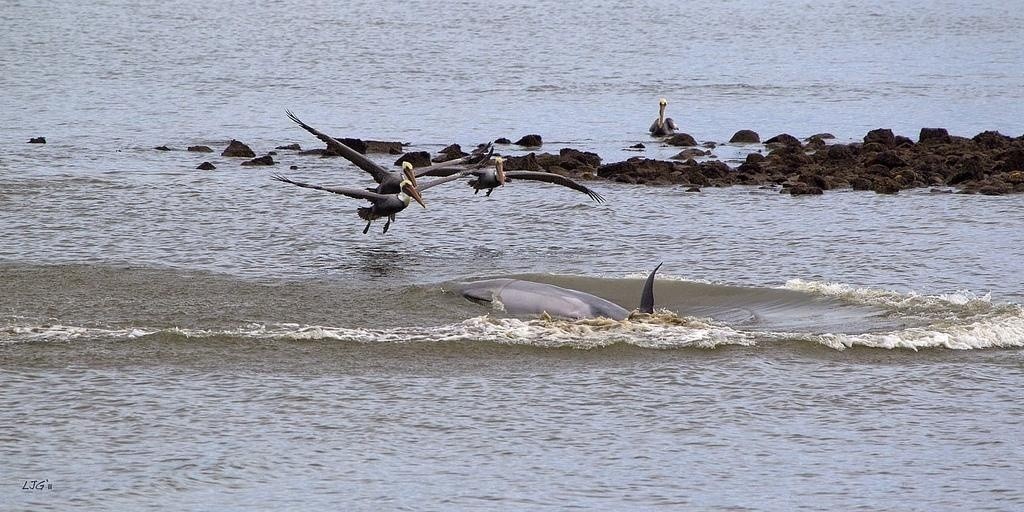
[267,145,495,234]
[649,97,682,137]
[282,106,493,194]
[384,154,609,207]
